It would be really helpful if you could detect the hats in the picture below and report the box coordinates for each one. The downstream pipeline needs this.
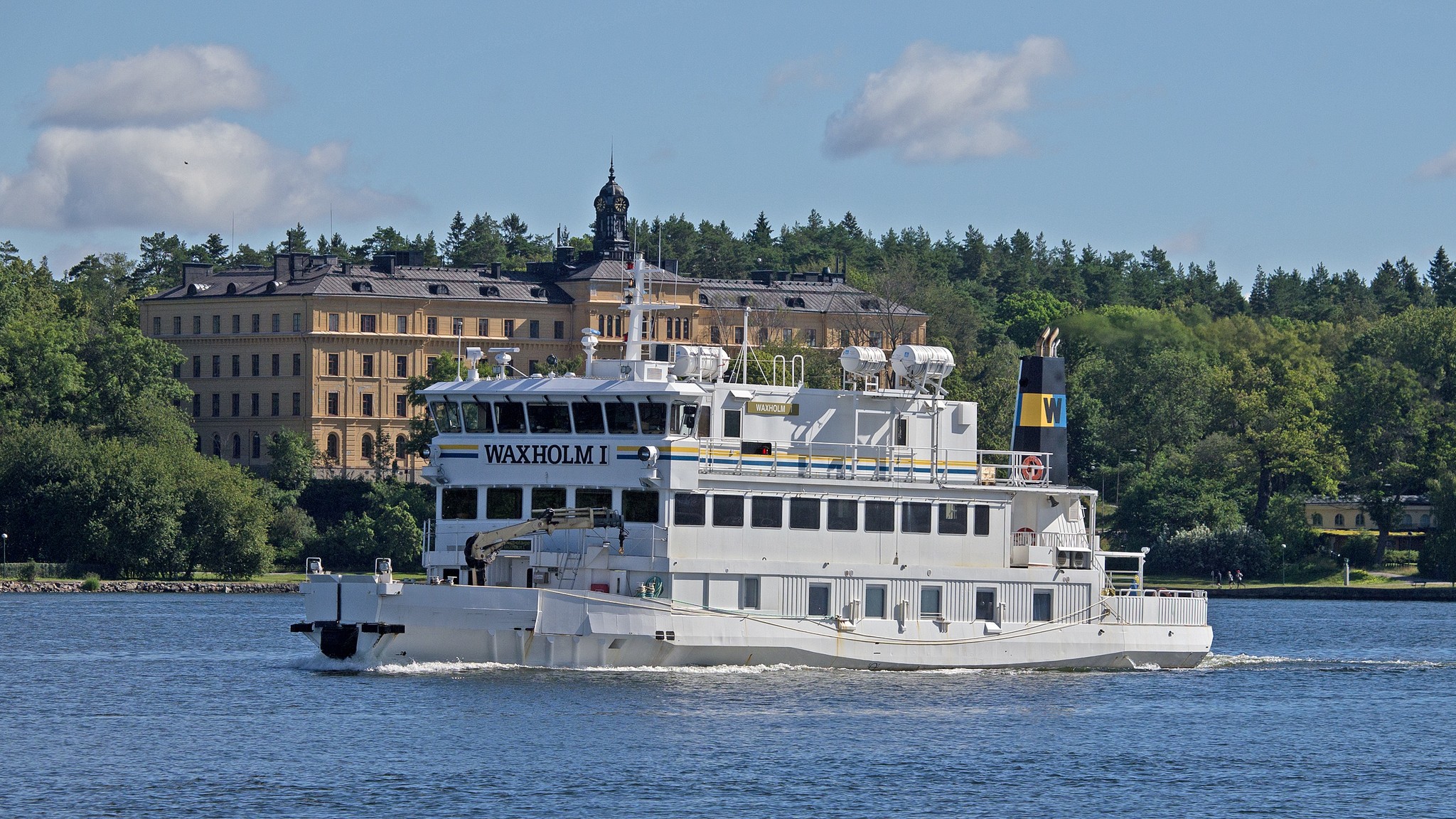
[1236,570,1240,572]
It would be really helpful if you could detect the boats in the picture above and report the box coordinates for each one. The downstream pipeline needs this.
[286,215,1210,676]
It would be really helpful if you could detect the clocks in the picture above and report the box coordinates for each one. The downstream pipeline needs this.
[595,197,605,212]
[615,197,626,212]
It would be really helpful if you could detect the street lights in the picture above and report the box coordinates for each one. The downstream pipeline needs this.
[1,534,7,579]
[1281,544,1286,586]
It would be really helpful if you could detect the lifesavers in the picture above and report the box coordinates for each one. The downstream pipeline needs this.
[1013,527,1036,546]
[1021,456,1043,480]
[1152,587,1172,597]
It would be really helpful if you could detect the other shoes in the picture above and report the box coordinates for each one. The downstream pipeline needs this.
[1233,582,1235,585]
[1230,587,1232,589]
[1218,586,1222,589]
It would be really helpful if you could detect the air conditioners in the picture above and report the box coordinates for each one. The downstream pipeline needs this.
[1057,550,1070,568]
[1070,552,1087,568]
